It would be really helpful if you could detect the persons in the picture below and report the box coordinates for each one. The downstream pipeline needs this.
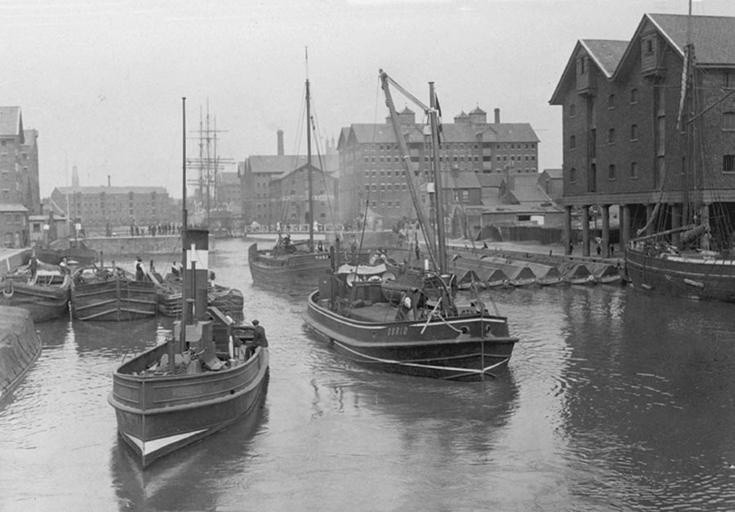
[172,260,183,276]
[279,221,291,231]
[370,248,394,268]
[136,256,146,280]
[400,291,412,314]
[315,240,325,259]
[130,222,182,235]
[392,215,420,242]
[210,272,216,288]
[349,235,359,252]
[244,320,269,361]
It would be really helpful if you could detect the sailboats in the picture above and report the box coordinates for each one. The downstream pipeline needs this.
[186,96,236,240]
[311,362,518,511]
[247,46,330,283]
[623,0,735,304]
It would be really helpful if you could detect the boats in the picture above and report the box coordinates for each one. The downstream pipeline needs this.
[300,67,520,384]
[33,314,70,348]
[107,96,268,465]
[24,224,59,265]
[108,405,268,511]
[69,318,158,359]
[71,251,156,322]
[0,249,71,323]
[59,219,99,265]
[160,314,243,330]
[157,261,243,317]
